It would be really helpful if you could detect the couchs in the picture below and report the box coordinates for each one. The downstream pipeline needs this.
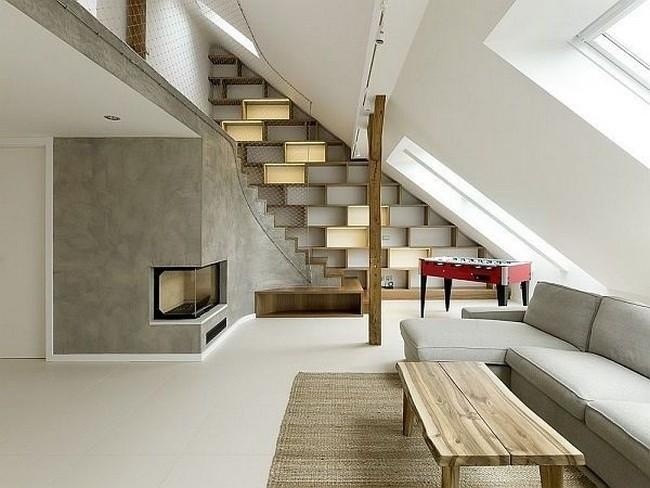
[400,280,650,488]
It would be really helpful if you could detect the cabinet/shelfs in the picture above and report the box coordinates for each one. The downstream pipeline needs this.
[208,54,496,289]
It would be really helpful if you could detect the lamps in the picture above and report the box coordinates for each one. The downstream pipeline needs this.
[362,10,386,114]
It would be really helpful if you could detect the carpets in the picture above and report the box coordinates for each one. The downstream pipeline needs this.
[266,371,596,488]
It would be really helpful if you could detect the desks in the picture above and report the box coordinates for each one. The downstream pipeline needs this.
[418,255,532,318]
